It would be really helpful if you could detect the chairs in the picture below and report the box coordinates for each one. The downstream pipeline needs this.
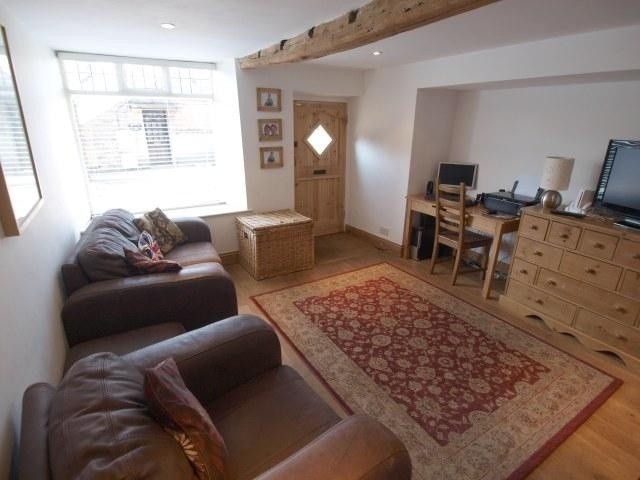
[430,177,493,285]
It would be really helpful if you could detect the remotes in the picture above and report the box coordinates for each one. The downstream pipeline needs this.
[550,210,586,219]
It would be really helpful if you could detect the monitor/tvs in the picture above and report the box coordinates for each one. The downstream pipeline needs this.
[437,162,478,194]
[590,139,640,232]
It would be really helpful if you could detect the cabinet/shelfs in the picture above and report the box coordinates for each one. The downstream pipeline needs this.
[498,203,640,376]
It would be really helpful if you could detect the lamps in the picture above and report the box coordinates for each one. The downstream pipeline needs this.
[541,156,575,215]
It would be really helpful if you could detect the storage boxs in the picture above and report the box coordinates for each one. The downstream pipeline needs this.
[236,207,315,281]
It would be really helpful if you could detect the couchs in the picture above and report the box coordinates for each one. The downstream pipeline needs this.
[61,208,238,350]
[18,312,412,480]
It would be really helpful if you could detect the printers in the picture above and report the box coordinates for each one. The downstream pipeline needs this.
[482,181,544,217]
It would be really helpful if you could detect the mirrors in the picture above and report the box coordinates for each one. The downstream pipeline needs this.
[302,120,336,160]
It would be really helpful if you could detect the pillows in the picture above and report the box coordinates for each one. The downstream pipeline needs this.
[132,207,187,254]
[139,356,232,480]
[122,245,182,274]
[137,230,164,261]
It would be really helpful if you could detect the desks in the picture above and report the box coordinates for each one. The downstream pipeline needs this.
[402,190,520,301]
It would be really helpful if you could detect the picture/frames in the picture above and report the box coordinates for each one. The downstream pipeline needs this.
[260,147,284,169]
[256,88,282,112]
[257,119,283,142]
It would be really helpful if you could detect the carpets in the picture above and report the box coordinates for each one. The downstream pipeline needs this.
[250,261,625,480]
